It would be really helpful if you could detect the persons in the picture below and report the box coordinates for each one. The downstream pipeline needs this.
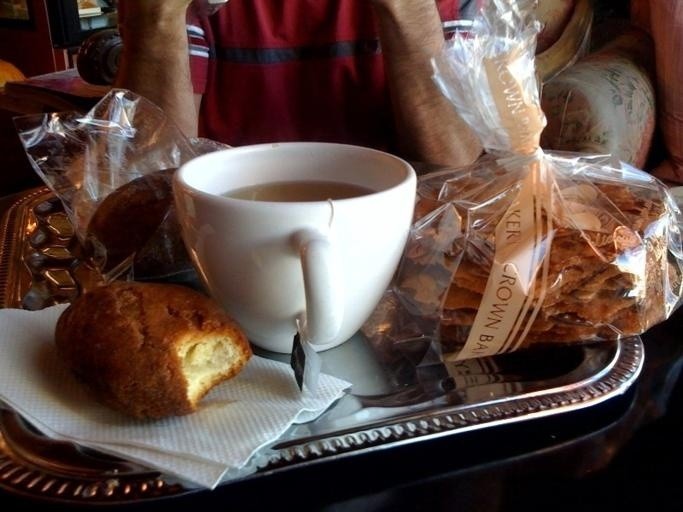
[110,0,485,172]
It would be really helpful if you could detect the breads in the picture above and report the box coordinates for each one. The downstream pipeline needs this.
[406,183,679,345]
[53,279,252,419]
[83,167,211,295]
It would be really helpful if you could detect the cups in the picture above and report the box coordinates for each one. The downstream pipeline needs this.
[173,141,417,354]
